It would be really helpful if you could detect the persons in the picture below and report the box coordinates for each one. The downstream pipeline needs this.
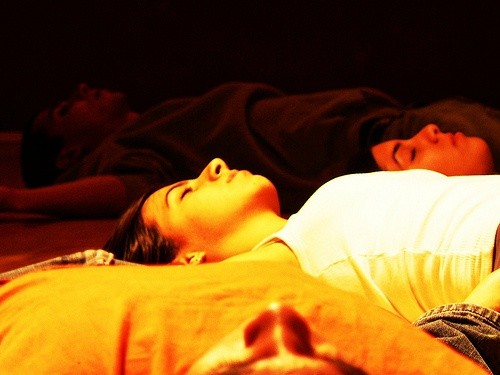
[0,80,500,219]
[0,246,500,375]
[343,120,500,176]
[102,156,500,326]
